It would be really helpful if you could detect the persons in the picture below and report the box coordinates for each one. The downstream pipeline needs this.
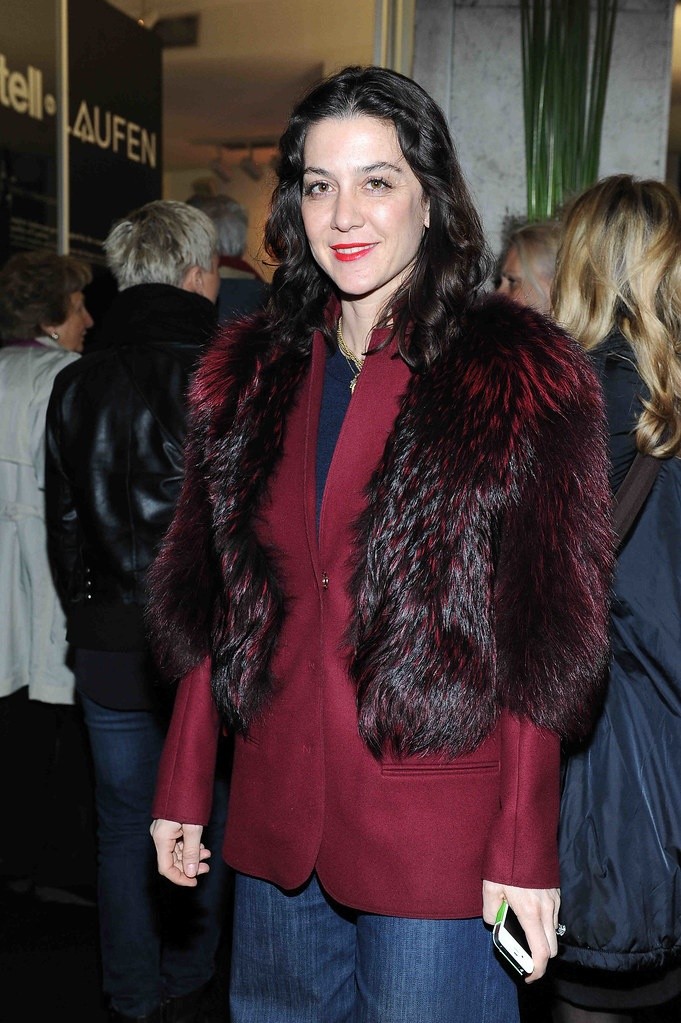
[1,191,271,1023]
[149,63,614,1023]
[495,173,681,1023]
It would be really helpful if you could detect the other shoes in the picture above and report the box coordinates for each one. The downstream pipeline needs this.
[7,871,34,893]
[38,873,99,908]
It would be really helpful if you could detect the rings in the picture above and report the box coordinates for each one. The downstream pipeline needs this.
[555,924,566,936]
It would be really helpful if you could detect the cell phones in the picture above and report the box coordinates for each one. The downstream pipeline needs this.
[492,899,534,976]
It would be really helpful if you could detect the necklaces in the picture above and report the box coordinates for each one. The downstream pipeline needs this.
[337,317,364,394]
